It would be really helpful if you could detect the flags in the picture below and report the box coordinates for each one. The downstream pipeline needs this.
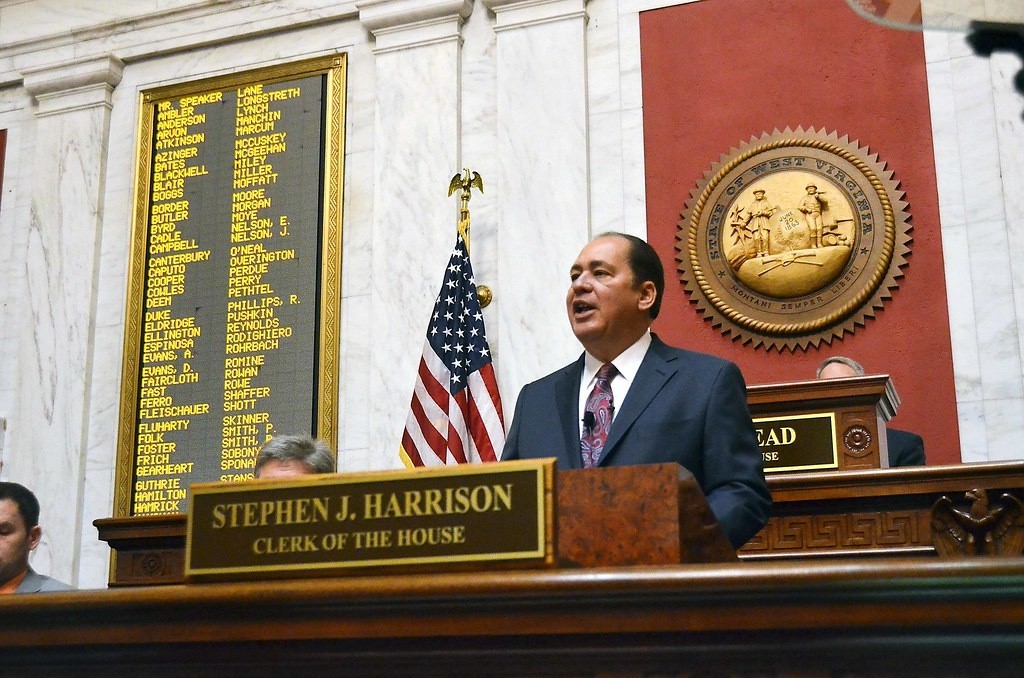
[398,220,505,470]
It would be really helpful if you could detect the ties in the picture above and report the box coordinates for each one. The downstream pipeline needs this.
[578,360,620,468]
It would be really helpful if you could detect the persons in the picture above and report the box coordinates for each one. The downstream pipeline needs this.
[740,188,774,258]
[499,232,772,553]
[798,182,828,248]
[0,482,78,594]
[256,432,334,480]
[817,355,929,465]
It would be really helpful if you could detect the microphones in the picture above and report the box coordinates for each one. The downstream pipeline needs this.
[583,411,594,467]
